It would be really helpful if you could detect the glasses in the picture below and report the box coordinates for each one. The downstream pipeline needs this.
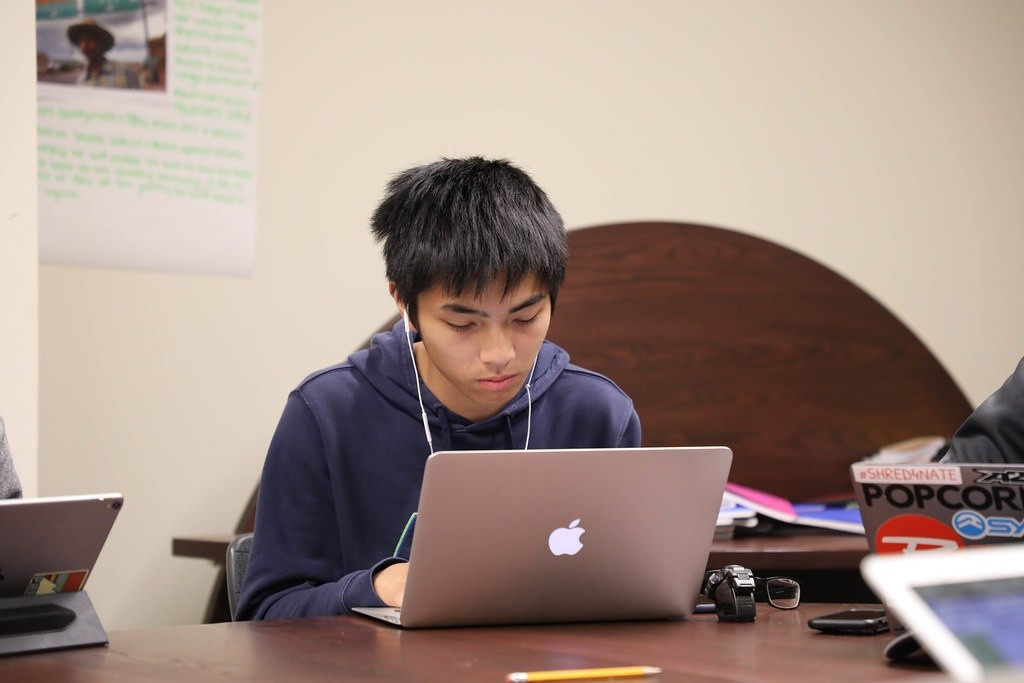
[705,569,804,610]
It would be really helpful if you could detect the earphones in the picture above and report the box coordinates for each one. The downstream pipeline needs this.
[404,304,411,333]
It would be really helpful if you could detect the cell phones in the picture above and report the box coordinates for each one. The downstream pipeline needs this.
[808,608,889,634]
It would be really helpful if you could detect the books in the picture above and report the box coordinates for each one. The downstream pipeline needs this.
[710,481,867,540]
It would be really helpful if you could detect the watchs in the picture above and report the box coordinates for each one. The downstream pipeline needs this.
[704,564,757,622]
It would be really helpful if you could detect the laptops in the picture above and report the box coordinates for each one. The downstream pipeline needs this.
[861,542,1023,683]
[350,446,734,630]
[848,461,1024,556]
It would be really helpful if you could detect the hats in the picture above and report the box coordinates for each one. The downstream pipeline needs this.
[67,18,115,53]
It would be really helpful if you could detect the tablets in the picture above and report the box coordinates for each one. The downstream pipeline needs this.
[0,494,123,596]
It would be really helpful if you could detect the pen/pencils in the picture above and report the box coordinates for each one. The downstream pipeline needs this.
[506,665,663,682]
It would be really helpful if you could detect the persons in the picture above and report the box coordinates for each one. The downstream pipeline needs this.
[233,151,641,623]
[66,18,139,90]
